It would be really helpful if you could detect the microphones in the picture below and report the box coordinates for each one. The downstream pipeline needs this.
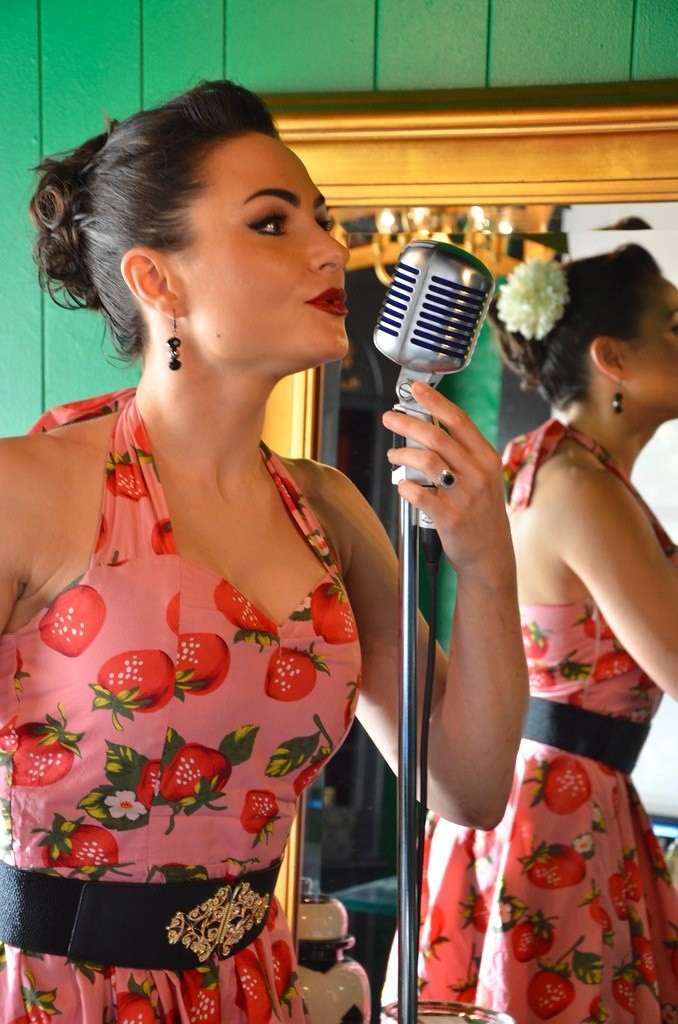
[372,239,496,560]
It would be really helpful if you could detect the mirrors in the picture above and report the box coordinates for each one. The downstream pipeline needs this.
[257,79,678,1024]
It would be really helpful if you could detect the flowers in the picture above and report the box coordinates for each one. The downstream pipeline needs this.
[494,255,571,340]
